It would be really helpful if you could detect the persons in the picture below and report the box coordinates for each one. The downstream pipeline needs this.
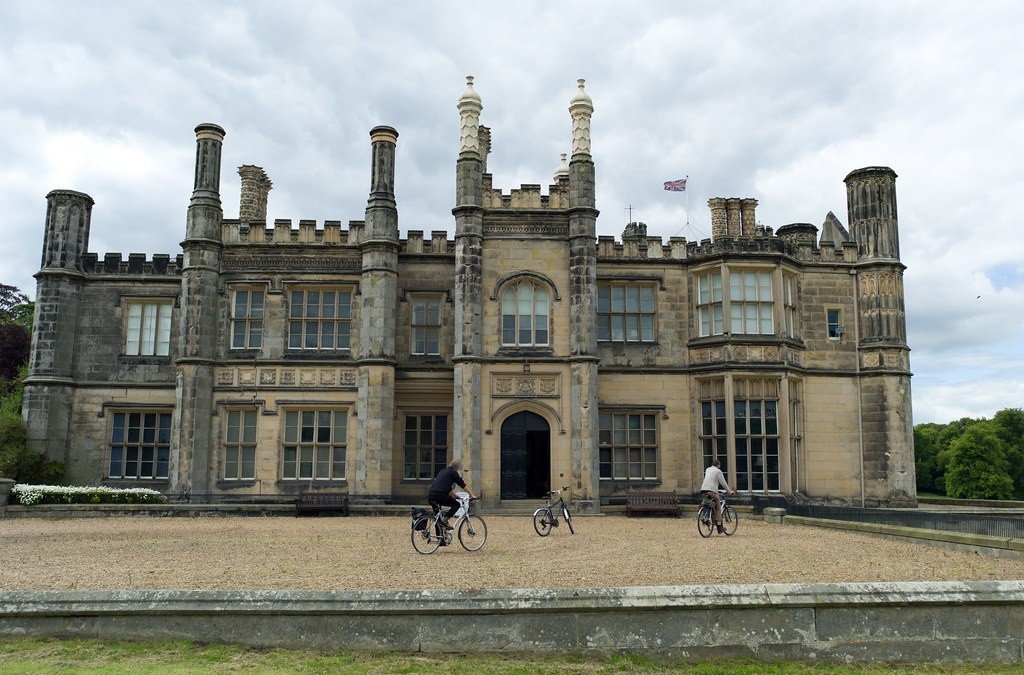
[700,459,732,534]
[428,459,478,546]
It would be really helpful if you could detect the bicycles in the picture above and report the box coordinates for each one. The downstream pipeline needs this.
[411,497,487,555]
[533,484,575,537]
[697,491,739,539]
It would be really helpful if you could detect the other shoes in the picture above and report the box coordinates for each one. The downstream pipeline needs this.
[441,520,453,530]
[439,540,450,546]
[717,525,726,533]
[702,517,709,525]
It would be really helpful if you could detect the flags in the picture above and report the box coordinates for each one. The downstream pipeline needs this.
[664,179,687,191]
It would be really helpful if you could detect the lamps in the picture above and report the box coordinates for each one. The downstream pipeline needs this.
[524,362,530,372]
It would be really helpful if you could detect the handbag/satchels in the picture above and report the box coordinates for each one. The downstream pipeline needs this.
[411,506,428,530]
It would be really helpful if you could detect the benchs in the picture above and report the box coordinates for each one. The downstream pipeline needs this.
[294,491,349,518]
[625,489,683,519]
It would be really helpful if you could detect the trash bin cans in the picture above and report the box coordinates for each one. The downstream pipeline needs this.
[455,492,470,517]
